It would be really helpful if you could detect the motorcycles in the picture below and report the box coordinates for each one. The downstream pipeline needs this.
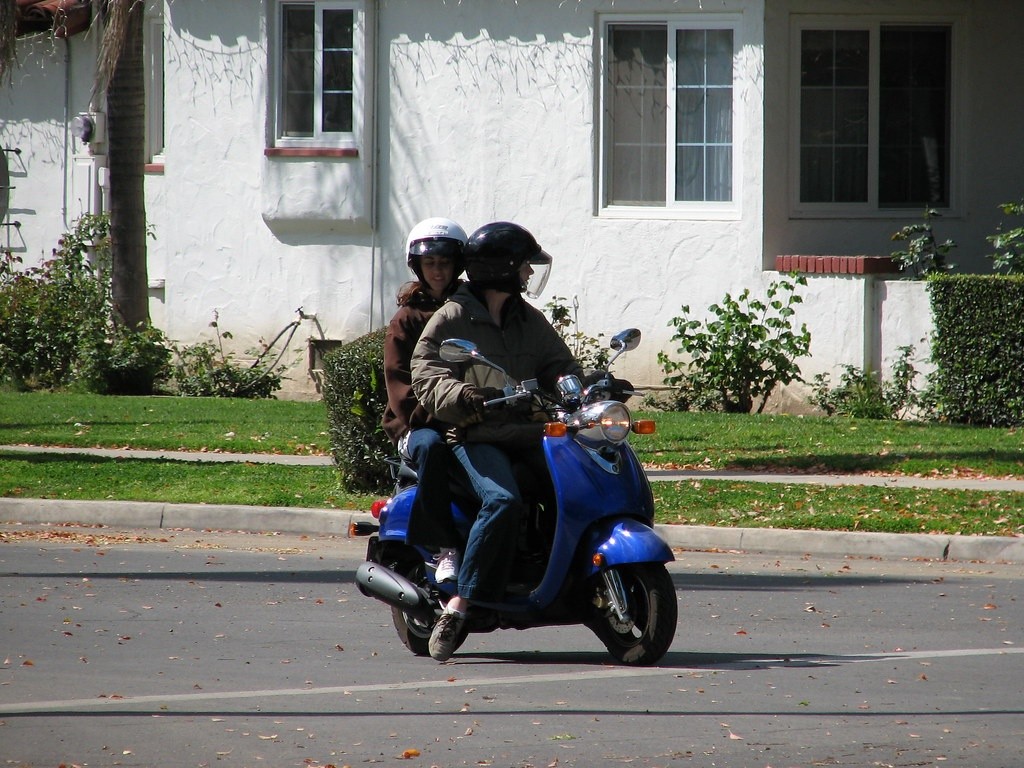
[348,327,680,667]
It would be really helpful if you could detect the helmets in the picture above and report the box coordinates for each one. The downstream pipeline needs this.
[405,216,468,282]
[462,222,553,300]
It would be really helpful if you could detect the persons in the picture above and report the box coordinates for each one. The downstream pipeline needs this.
[381,219,471,582]
[414,222,636,663]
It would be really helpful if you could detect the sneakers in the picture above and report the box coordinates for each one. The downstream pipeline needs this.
[428,609,466,662]
[435,547,461,585]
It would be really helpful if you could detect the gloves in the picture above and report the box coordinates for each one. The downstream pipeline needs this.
[604,378,635,403]
[471,387,507,418]
